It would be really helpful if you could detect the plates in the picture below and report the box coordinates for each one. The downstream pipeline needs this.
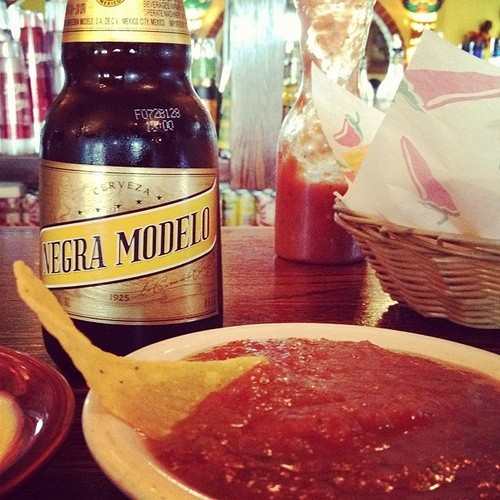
[0,346,75,500]
[81,321,500,500]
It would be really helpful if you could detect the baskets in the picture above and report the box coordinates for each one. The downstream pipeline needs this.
[333,191,500,329]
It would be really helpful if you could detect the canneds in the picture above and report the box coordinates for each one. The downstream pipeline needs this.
[219,182,276,227]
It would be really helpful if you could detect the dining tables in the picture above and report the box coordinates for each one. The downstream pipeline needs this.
[0,226,500,500]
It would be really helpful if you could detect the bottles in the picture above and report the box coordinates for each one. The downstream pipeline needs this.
[191,38,220,135]
[274,0,377,262]
[0,0,62,156]
[38,0,224,378]
